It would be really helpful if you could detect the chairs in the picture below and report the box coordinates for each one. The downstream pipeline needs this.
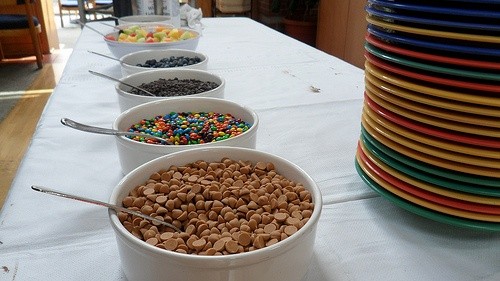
[0,0,43,69]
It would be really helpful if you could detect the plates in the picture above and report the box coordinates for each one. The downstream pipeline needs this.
[354,1,500,232]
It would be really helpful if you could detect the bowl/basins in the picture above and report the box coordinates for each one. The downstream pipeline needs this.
[113,96,258,177]
[108,146,322,281]
[104,28,200,58]
[120,50,208,78]
[113,15,174,32]
[116,70,225,113]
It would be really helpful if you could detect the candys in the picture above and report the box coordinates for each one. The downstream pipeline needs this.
[124,112,250,145]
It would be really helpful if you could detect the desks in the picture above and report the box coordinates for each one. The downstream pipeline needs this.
[0,17,500,281]
